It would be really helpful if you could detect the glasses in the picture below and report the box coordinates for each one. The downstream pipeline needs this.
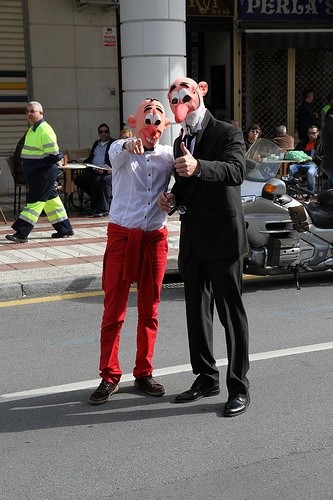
[163,193,189,214]
[308,132,317,135]
[98,130,109,133]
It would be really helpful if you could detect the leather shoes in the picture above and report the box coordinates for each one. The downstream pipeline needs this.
[174,378,220,403]
[224,389,250,416]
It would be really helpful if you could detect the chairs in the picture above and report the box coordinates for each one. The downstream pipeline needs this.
[67,148,91,210]
[7,155,29,214]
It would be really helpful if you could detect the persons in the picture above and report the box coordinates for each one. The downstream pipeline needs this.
[245,124,262,152]
[5,101,74,243]
[158,77,250,416]
[273,125,294,148]
[79,124,134,217]
[89,98,175,405]
[288,90,333,199]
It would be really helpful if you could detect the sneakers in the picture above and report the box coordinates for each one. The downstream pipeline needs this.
[89,379,119,405]
[51,229,74,239]
[5,233,28,243]
[134,375,166,396]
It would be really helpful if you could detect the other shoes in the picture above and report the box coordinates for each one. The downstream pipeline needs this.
[94,209,110,217]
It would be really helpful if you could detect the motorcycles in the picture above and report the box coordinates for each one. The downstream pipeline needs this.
[237,137,333,290]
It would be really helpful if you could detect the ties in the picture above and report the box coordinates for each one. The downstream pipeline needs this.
[185,132,195,151]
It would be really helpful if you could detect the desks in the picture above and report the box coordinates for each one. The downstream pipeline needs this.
[262,159,299,176]
[57,163,88,218]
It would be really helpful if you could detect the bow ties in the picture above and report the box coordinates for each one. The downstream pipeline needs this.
[142,146,154,151]
